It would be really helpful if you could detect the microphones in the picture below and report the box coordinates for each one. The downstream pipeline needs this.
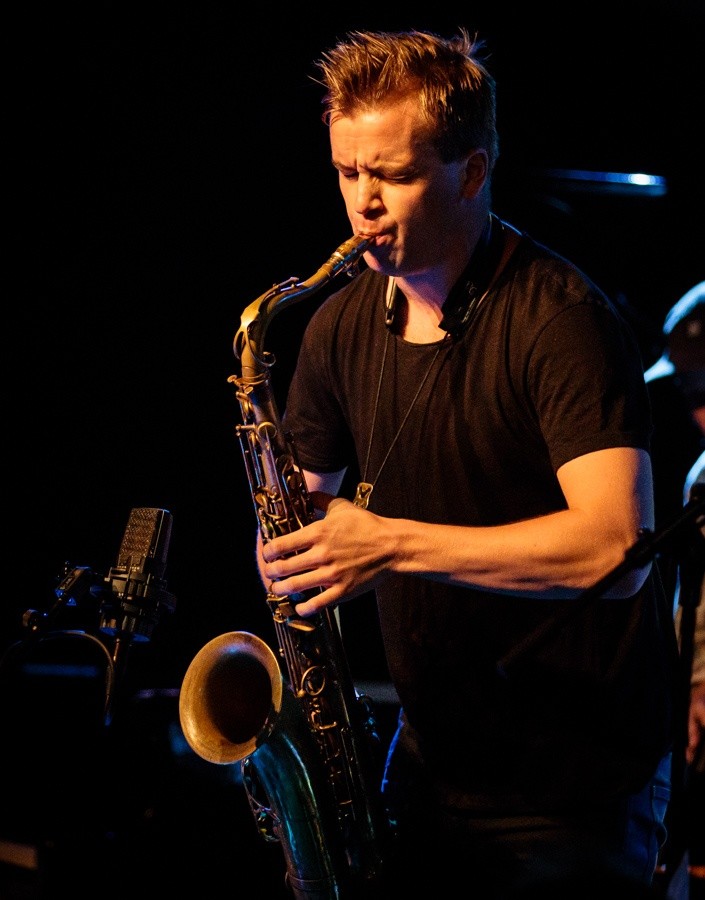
[106,507,174,662]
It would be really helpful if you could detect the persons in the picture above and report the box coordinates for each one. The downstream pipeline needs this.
[256,29,683,900]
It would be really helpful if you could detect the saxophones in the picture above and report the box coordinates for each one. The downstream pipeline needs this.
[177,229,401,900]
